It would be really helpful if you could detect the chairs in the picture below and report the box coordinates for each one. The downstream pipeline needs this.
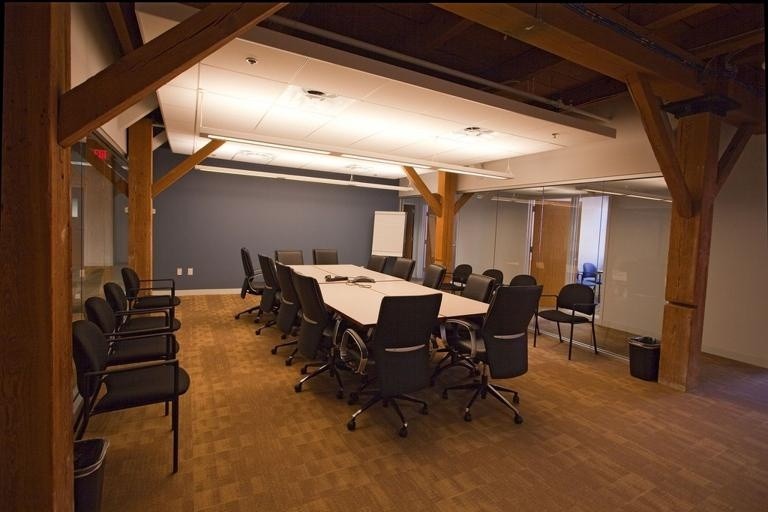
[577,263,602,295]
[85,296,180,416]
[534,283,598,360]
[121,267,180,320]
[72,320,190,473]
[104,282,181,338]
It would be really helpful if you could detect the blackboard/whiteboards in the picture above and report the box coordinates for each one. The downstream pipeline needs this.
[371,210,407,258]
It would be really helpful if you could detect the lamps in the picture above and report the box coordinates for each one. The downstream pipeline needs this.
[576,182,672,203]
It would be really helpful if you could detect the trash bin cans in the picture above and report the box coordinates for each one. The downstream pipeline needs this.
[72,438,111,511]
[628,336,661,382]
